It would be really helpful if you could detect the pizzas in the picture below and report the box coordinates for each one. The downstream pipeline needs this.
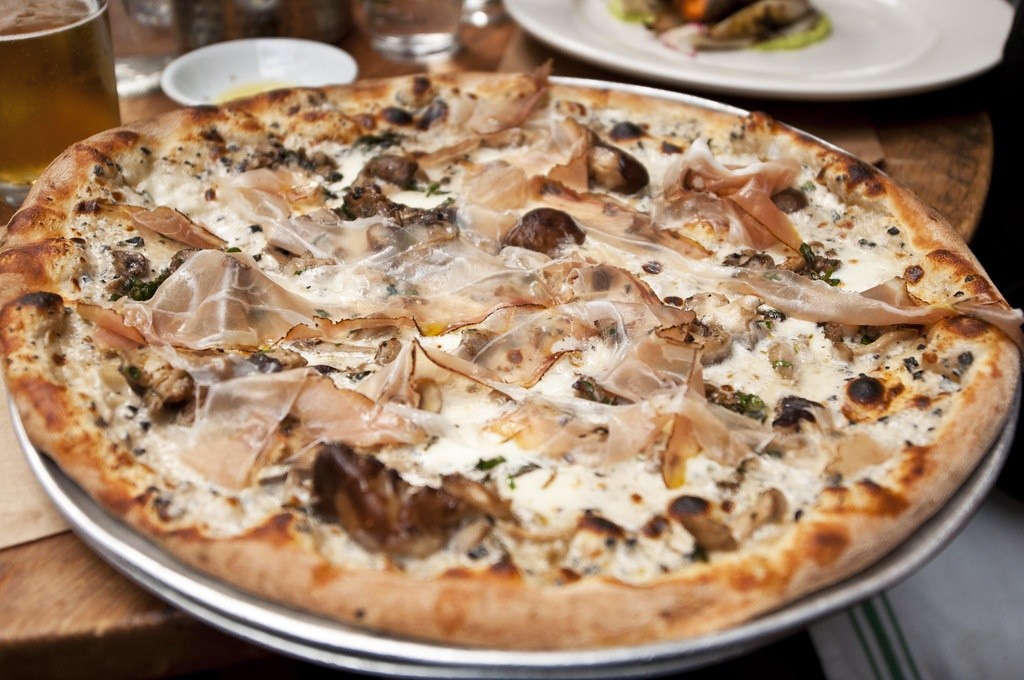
[0,57,1024,654]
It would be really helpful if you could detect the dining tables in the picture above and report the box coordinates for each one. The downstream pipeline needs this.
[0,0,994,679]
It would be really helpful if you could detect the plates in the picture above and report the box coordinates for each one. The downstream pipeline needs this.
[160,37,360,110]
[499,0,1015,123]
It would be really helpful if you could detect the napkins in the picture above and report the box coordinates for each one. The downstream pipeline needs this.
[0,374,70,550]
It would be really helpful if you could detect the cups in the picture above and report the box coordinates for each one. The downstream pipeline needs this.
[103,0,181,98]
[363,0,462,64]
[0,0,125,213]
